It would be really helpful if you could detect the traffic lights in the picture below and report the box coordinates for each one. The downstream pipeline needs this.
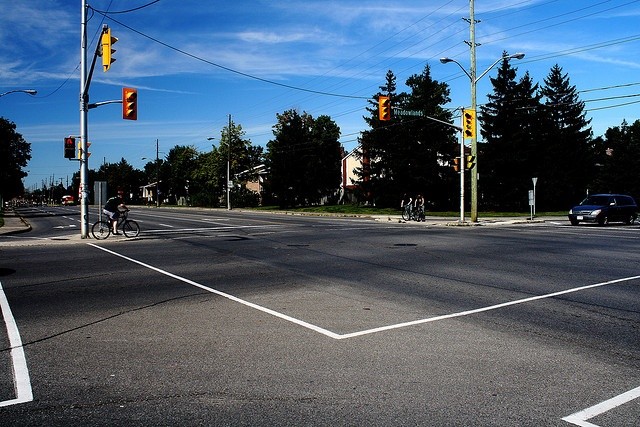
[123,87,137,120]
[79,142,91,159]
[464,155,476,169]
[452,158,460,172]
[379,96,391,120]
[102,29,119,72]
[64,137,75,158]
[463,109,476,138]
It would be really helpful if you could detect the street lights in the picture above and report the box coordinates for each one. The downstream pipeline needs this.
[439,52,526,221]
[206,137,231,209]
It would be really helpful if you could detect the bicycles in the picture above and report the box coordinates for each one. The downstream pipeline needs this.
[401,204,411,221]
[91,210,140,240]
[412,205,426,222]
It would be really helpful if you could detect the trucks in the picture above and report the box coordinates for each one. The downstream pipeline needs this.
[61,195,74,206]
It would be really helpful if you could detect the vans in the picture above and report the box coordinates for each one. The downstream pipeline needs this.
[568,194,638,226]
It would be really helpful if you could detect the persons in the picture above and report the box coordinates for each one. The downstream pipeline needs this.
[414,193,426,216]
[400,192,413,219]
[102,189,131,236]
